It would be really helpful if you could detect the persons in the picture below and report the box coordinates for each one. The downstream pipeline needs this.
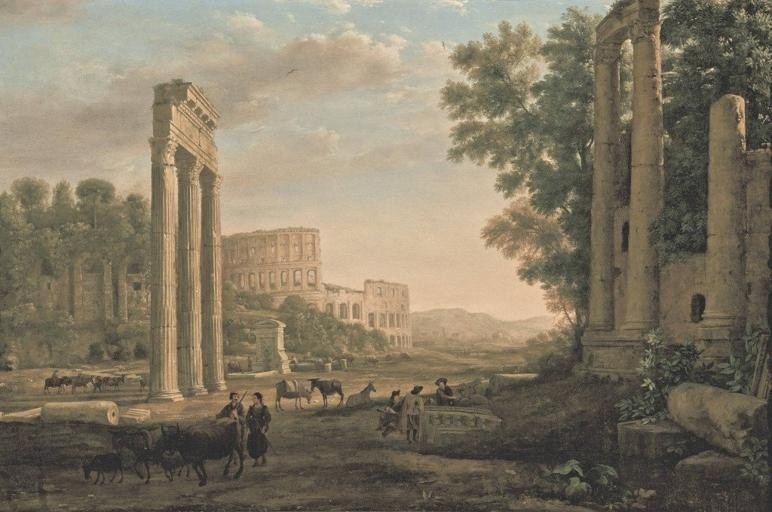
[216,392,246,464]
[246,393,272,467]
[435,378,456,407]
[376,391,403,436]
[404,385,425,443]
[52,370,59,388]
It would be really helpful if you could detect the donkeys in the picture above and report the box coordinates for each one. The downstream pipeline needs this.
[79,417,245,487]
[43,373,125,394]
[272,378,312,409]
[307,378,344,408]
[346,380,376,408]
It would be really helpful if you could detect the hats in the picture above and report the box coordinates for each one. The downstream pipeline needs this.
[435,377,447,385]
[411,385,423,394]
[391,390,400,399]
